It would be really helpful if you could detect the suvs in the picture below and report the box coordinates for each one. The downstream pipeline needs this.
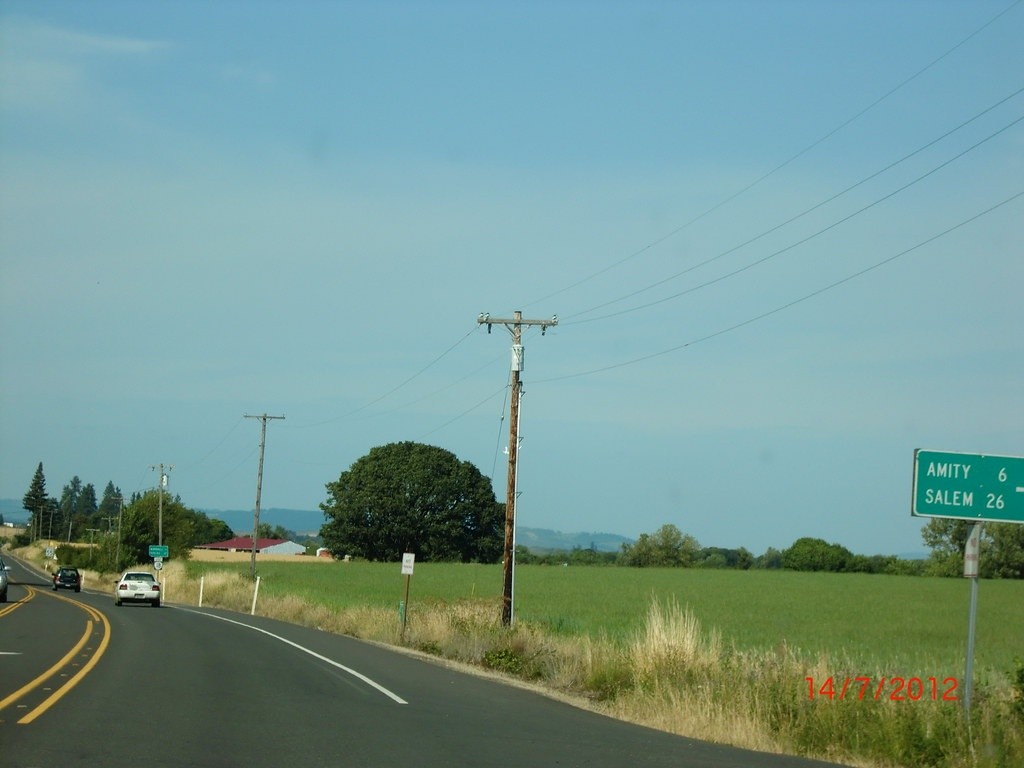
[114,573,161,607]
[0,557,12,603]
[50,567,81,592]
